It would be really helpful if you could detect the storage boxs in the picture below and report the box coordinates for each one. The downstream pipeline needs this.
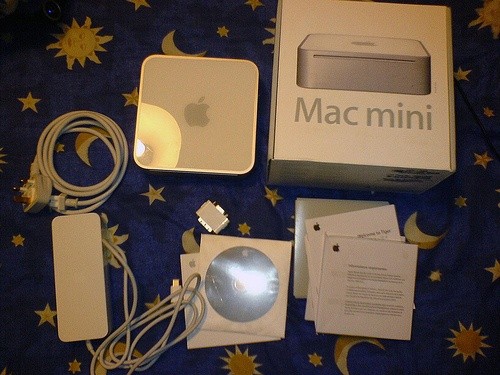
[265,0,457,194]
